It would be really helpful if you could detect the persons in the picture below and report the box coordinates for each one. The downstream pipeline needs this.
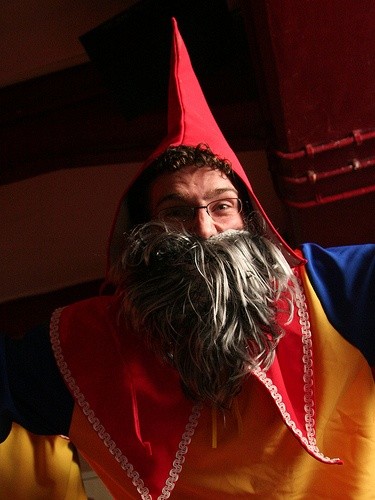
[1,144,375,500]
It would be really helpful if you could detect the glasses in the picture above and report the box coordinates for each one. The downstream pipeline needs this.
[151,196,243,224]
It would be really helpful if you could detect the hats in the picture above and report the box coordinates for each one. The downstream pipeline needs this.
[108,17,308,270]
